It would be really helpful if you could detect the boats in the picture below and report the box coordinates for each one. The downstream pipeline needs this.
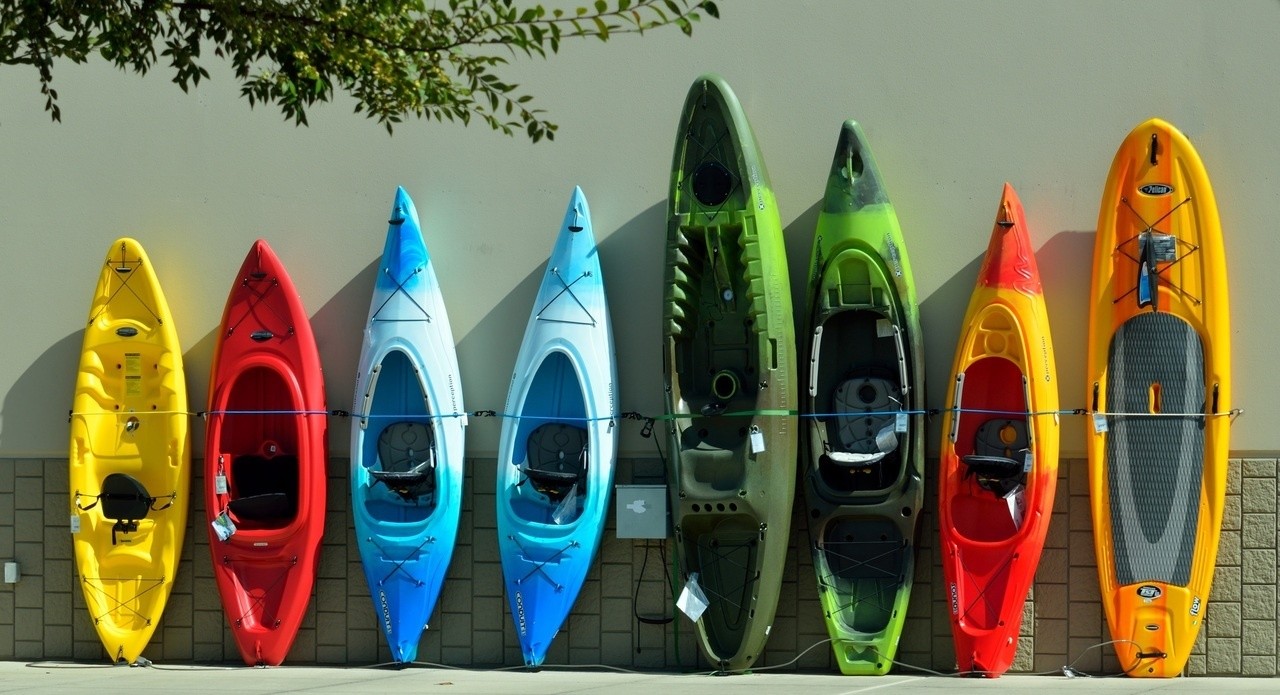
[800,121,926,678]
[1087,119,1230,678]
[350,186,465,663]
[69,238,190,666]
[205,240,327,666]
[938,185,1059,679]
[496,185,620,672]
[661,73,798,675]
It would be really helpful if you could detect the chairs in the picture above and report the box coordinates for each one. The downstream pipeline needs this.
[822,375,899,486]
[679,403,749,497]
[95,472,153,524]
[228,451,299,522]
[963,418,1028,491]
[369,420,436,497]
[524,423,588,500]
